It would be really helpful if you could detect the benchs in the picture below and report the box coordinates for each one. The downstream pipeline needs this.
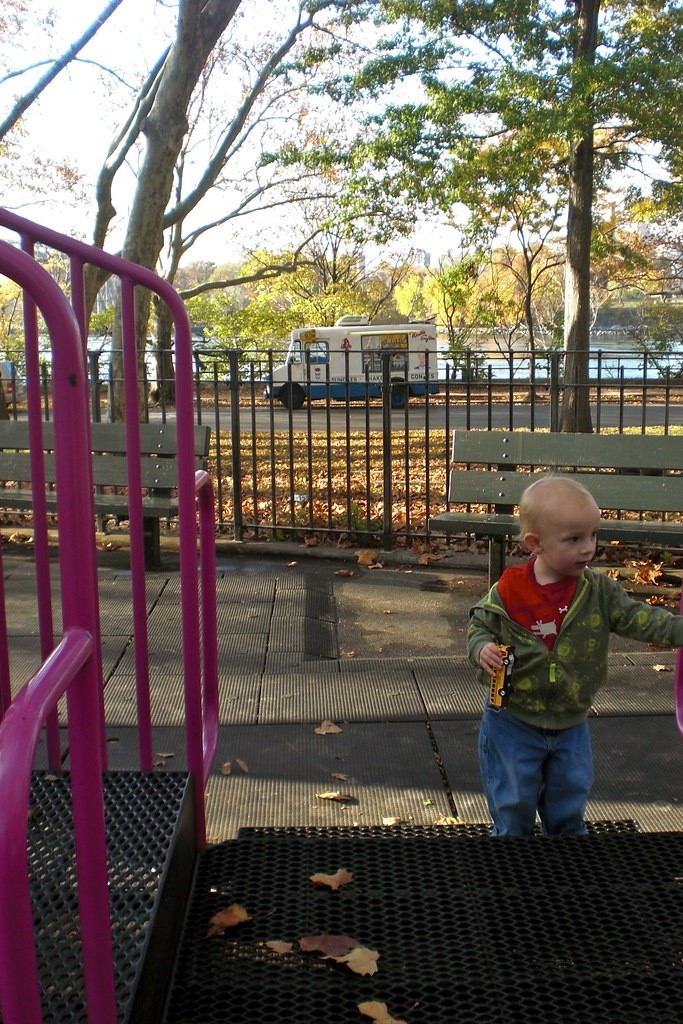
[0,419,212,566]
[425,428,683,591]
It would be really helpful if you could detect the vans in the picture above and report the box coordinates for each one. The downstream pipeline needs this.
[258,315,439,409]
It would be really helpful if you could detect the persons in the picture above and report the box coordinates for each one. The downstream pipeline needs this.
[467,476,683,836]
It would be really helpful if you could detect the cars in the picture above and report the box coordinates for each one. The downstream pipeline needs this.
[0,361,25,406]
